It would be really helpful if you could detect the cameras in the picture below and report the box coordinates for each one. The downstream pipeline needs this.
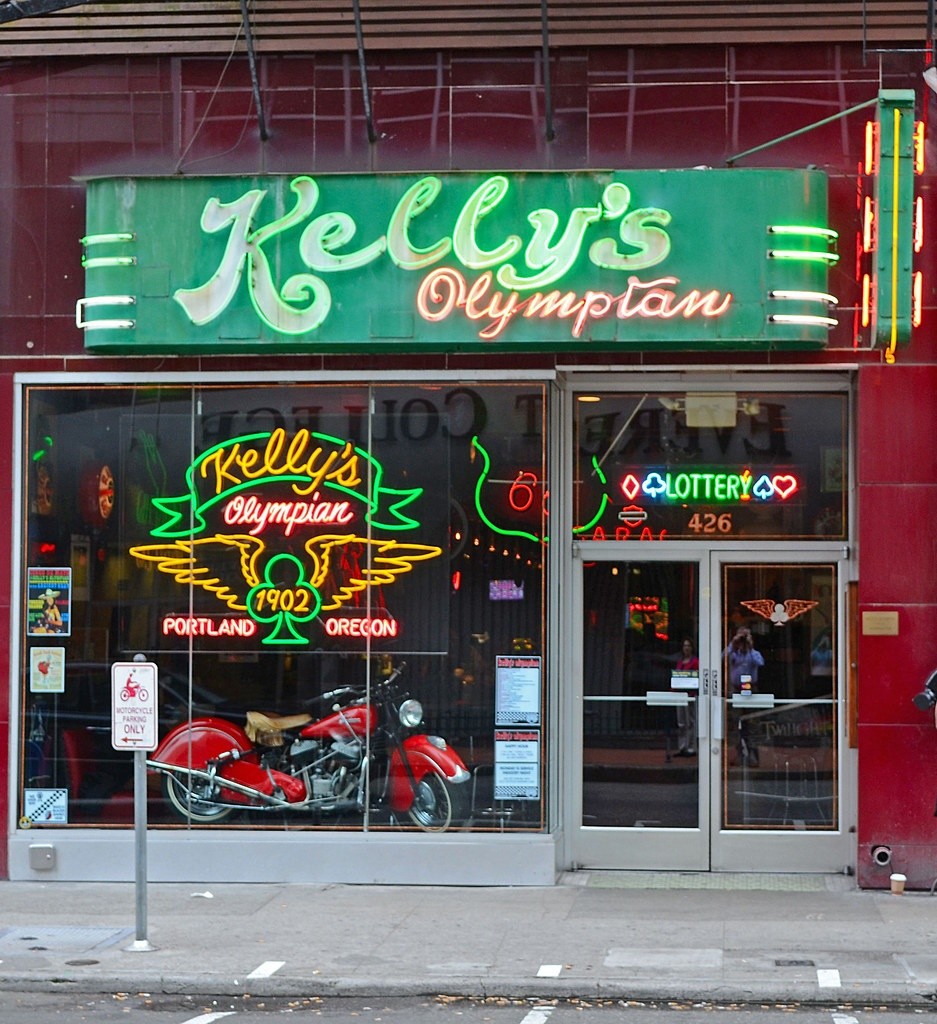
[739,636,748,642]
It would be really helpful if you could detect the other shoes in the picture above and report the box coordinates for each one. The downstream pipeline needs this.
[747,752,759,768]
[673,747,696,758]
[736,750,747,768]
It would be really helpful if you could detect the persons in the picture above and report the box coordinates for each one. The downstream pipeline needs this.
[811,635,835,714]
[674,637,699,757]
[722,627,763,770]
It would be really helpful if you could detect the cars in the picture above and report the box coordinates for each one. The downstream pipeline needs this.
[25,659,282,783]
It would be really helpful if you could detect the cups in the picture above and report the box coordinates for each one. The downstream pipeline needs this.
[890,874,907,896]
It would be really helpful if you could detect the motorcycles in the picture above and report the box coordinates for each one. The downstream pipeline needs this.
[143,661,473,833]
